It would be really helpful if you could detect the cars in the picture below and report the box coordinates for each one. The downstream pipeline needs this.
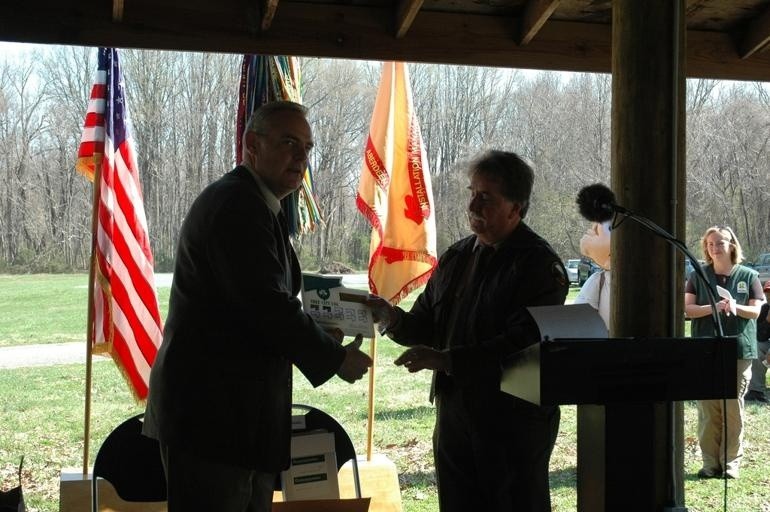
[685,260,709,282]
[565,259,598,284]
[745,254,770,405]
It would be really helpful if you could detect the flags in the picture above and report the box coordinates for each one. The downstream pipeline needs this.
[74,46,164,410]
[355,60,439,307]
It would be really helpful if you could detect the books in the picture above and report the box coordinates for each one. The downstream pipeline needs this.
[279,426,340,503]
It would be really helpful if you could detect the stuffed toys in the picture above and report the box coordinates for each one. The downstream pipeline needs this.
[573,221,612,336]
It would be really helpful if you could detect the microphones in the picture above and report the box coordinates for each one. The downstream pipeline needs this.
[577,184,725,336]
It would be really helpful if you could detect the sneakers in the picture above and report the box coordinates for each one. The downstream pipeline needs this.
[721,468,740,479]
[697,466,720,478]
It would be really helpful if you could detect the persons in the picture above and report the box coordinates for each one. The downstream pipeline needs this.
[743,280,770,403]
[140,99,374,512]
[684,225,765,481]
[362,148,570,512]
